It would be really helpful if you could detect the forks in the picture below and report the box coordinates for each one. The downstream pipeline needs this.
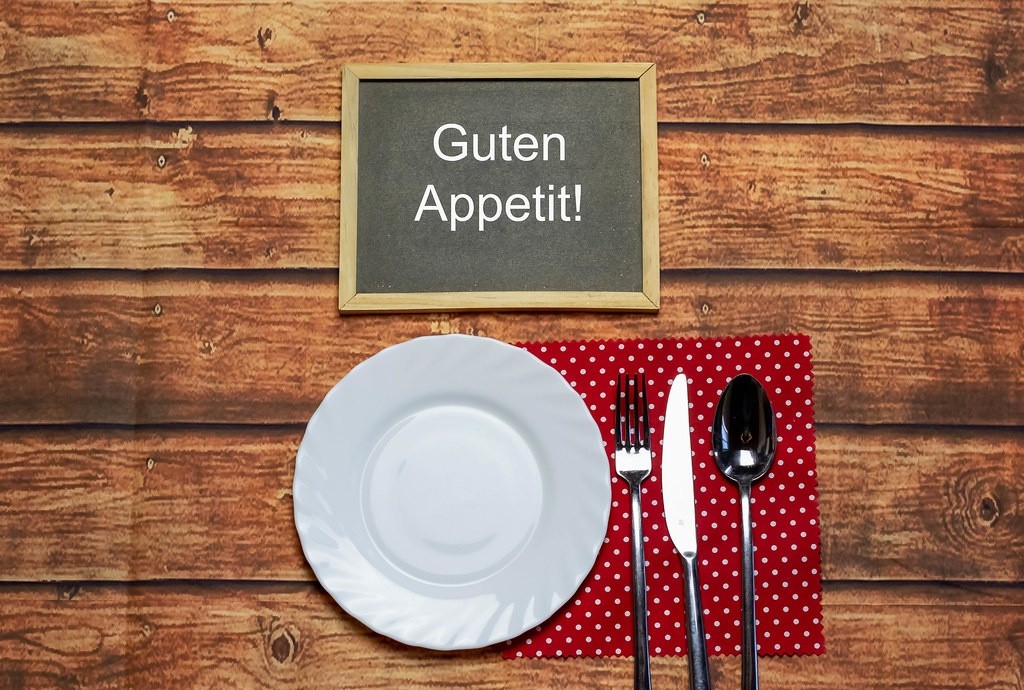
[614,371,656,690]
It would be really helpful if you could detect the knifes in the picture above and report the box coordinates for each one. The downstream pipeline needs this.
[661,374,712,689]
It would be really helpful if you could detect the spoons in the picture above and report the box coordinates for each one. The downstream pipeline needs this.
[712,372,776,690]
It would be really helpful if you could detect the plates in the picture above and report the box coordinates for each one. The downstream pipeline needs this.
[293,332,614,652]
